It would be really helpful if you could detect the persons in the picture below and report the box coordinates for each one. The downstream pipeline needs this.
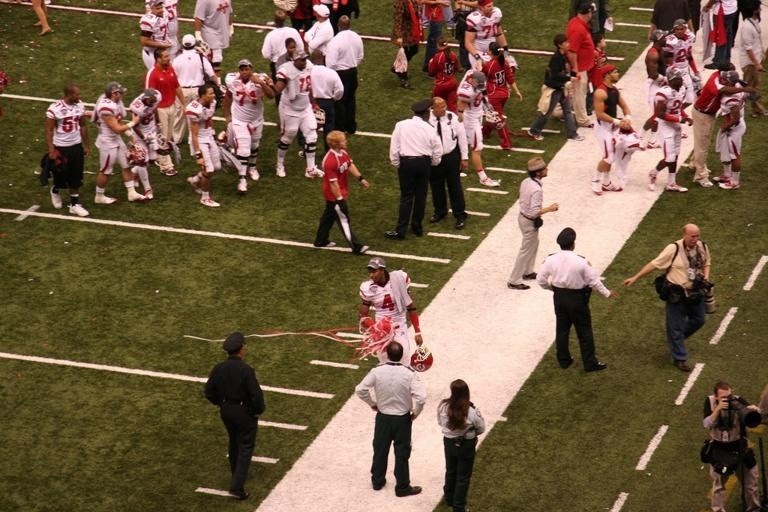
[537,227,617,369]
[507,157,559,290]
[315,132,370,253]
[702,382,765,512]
[640,1,768,191]
[437,379,485,512]
[358,258,423,364]
[46,1,364,217]
[385,1,523,239]
[528,0,646,195]
[32,0,51,36]
[354,341,427,497]
[205,332,265,499]
[625,223,710,369]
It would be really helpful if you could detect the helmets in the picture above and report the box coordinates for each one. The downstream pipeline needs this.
[410,344,433,372]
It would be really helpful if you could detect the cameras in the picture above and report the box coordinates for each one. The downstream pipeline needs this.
[692,272,716,292]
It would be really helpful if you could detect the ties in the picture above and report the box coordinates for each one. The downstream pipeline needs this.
[437,117,442,142]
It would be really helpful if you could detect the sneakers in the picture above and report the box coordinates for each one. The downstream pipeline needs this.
[50,186,153,217]
[523,272,537,280]
[304,165,323,178]
[480,176,500,187]
[187,168,259,208]
[160,168,178,176]
[601,180,623,192]
[646,162,741,193]
[527,130,544,141]
[507,282,530,289]
[359,245,370,253]
[567,134,586,141]
[591,173,603,195]
[276,164,286,177]
[314,241,336,247]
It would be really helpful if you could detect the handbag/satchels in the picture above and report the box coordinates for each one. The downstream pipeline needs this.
[653,275,686,305]
[205,80,223,109]
[705,444,740,476]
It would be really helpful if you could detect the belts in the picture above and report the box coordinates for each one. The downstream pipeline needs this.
[520,211,534,222]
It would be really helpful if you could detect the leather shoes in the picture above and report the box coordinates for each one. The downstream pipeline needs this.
[585,361,606,372]
[396,486,422,497]
[704,63,721,69]
[675,359,690,372]
[384,213,465,240]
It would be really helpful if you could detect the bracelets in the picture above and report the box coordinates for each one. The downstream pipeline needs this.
[614,119,620,125]
[624,115,630,119]
[358,176,364,182]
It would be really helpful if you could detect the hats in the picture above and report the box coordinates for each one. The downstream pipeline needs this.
[720,70,740,85]
[313,4,331,18]
[292,49,310,61]
[366,257,387,270]
[528,157,548,172]
[221,331,247,356]
[142,88,157,102]
[149,0,165,8]
[182,34,197,48]
[651,29,670,42]
[409,99,434,114]
[555,226,576,247]
[599,64,619,77]
[237,58,253,68]
[105,82,127,96]
[671,19,686,33]
